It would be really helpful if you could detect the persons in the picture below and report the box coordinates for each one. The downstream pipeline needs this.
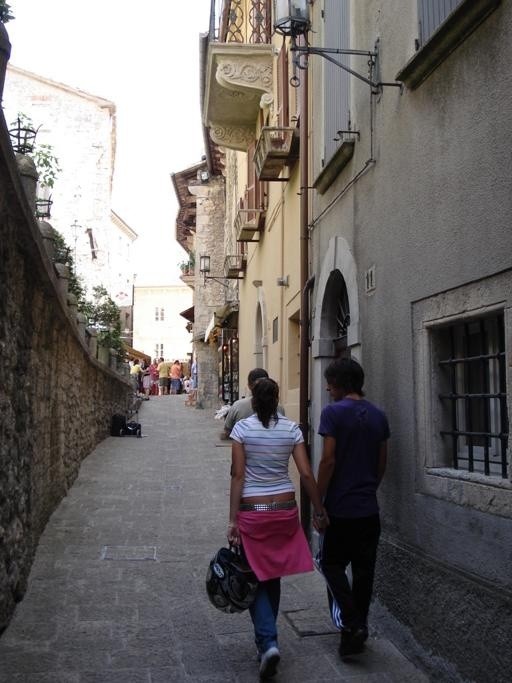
[226,377,327,678]
[225,368,286,439]
[314,358,391,656]
[129,357,191,395]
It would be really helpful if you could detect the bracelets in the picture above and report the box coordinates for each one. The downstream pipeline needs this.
[316,512,323,516]
[228,524,240,529]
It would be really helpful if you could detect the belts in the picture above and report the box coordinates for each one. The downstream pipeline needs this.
[238,500,297,512]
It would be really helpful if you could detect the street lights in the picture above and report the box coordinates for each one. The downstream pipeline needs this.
[71,219,82,280]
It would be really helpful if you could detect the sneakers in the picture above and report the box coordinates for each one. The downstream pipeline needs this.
[260,646,280,680]
[339,622,368,657]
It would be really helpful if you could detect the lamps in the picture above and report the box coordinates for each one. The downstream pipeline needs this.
[273,0,384,104]
[200,251,243,285]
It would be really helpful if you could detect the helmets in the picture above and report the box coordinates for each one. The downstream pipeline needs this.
[205,547,259,613]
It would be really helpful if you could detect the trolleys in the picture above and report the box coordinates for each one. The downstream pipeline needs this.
[111,391,150,437]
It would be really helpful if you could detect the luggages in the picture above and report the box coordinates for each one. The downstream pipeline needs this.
[111,413,126,435]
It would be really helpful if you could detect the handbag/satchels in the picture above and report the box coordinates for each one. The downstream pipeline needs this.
[126,420,141,434]
